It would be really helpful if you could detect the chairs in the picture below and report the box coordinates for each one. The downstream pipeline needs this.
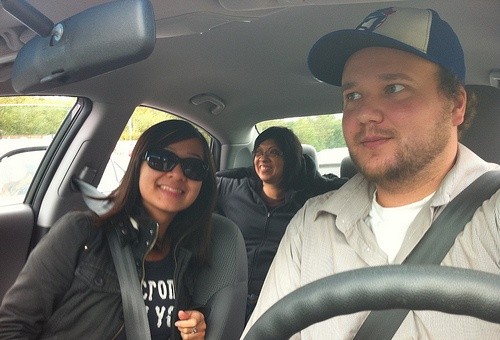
[190,212,249,340]
[214,144,317,179]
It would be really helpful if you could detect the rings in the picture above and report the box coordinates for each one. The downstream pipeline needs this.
[192,327,197,334]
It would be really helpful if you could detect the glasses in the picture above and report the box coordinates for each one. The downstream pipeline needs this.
[141,148,210,181]
[253,147,284,158]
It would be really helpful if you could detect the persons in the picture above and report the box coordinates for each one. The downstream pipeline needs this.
[217,126,350,329]
[0,119,217,340]
[240,5,500,340]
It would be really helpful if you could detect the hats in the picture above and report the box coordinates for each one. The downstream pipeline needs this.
[307,7,465,86]
